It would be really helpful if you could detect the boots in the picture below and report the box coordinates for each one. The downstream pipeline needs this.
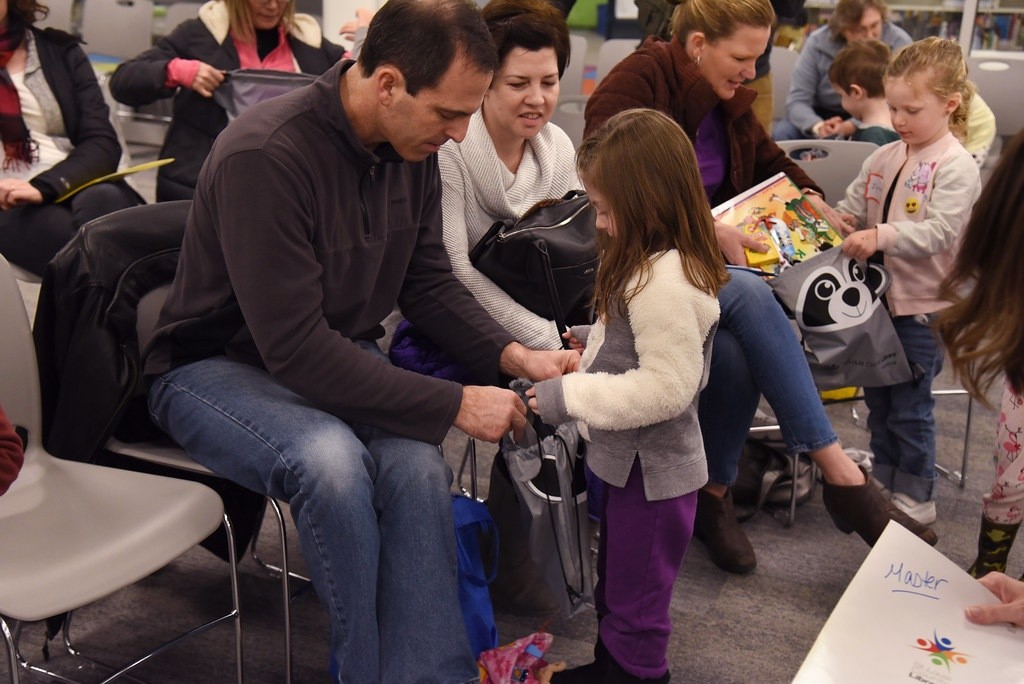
[964,512,1022,581]
[819,464,941,553]
[694,478,760,577]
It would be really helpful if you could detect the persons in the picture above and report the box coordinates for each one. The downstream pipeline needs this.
[583,0,939,573]
[949,91,997,167]
[0,0,147,277]
[109,1,374,202]
[965,571,1024,628]
[773,0,912,140]
[140,1,579,684]
[438,0,588,350]
[525,106,731,684]
[834,36,982,526]
[934,126,1024,579]
[829,39,901,146]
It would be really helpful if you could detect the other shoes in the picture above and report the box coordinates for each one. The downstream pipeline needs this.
[883,490,938,526]
[550,637,671,684]
[868,477,890,500]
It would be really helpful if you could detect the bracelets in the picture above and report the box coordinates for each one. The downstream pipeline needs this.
[800,188,822,197]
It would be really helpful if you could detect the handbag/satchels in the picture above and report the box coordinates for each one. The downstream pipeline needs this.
[487,385,597,630]
[211,67,320,128]
[736,435,816,506]
[771,241,910,390]
[439,436,499,665]
[470,188,602,350]
[475,633,562,684]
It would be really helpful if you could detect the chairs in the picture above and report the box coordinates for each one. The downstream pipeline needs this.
[0,36,1024,684]
